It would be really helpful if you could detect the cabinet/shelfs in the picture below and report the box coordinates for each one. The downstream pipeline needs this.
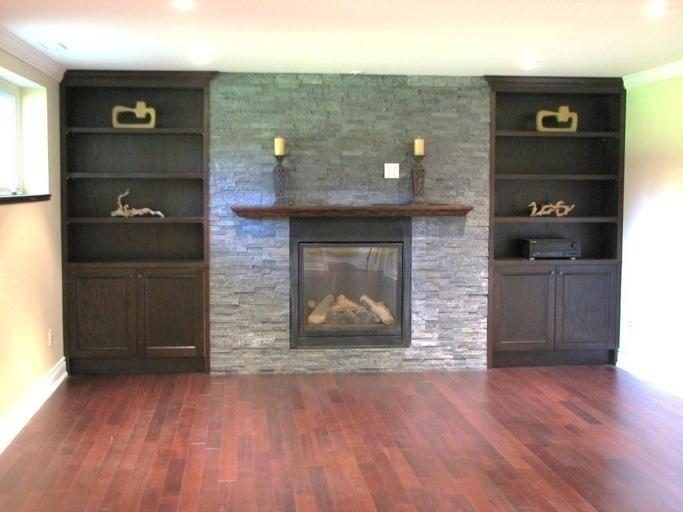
[487,76,625,367]
[59,70,214,378]
